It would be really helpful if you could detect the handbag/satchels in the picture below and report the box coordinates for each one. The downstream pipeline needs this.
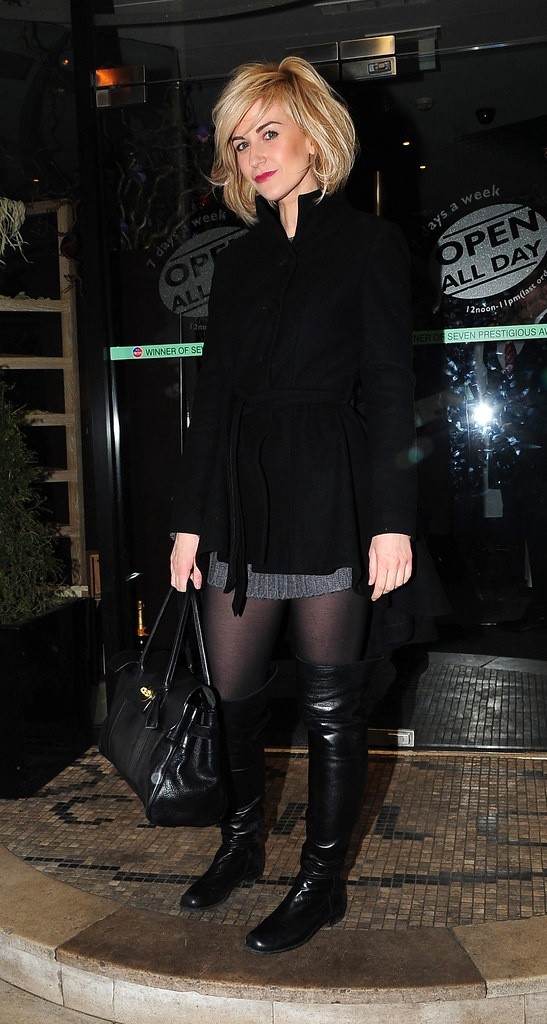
[97,576,229,828]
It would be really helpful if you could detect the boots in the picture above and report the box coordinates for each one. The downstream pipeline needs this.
[179,662,279,910]
[246,646,384,954]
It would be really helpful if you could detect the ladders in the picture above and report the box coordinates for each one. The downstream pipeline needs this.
[0,198,91,605]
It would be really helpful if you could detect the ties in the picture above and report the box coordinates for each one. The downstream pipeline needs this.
[505,342,517,375]
[482,341,504,394]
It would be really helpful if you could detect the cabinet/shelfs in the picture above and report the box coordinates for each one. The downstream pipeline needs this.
[0,198,91,599]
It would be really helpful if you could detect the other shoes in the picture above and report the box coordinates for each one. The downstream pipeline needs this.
[499,612,546,633]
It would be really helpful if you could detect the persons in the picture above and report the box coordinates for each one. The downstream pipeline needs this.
[413,279,547,633]
[167,56,452,955]
